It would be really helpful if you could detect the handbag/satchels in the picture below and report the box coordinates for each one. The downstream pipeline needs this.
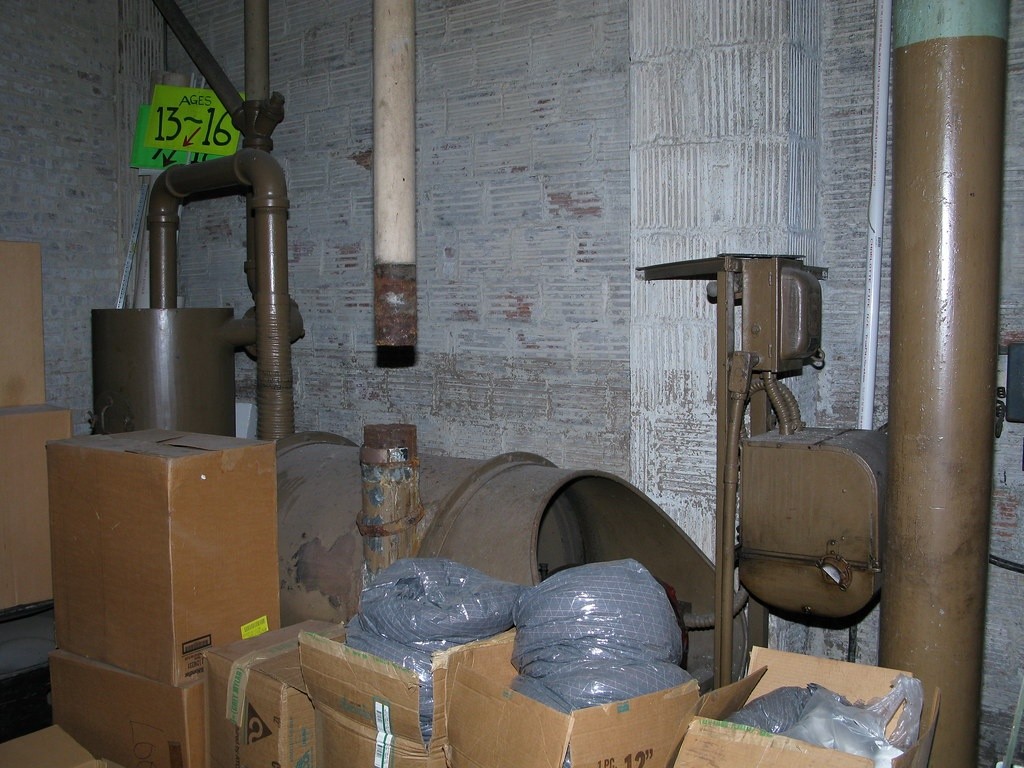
[779,673,924,768]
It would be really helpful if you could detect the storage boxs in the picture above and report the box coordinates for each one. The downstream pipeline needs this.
[0,244,939,768]
